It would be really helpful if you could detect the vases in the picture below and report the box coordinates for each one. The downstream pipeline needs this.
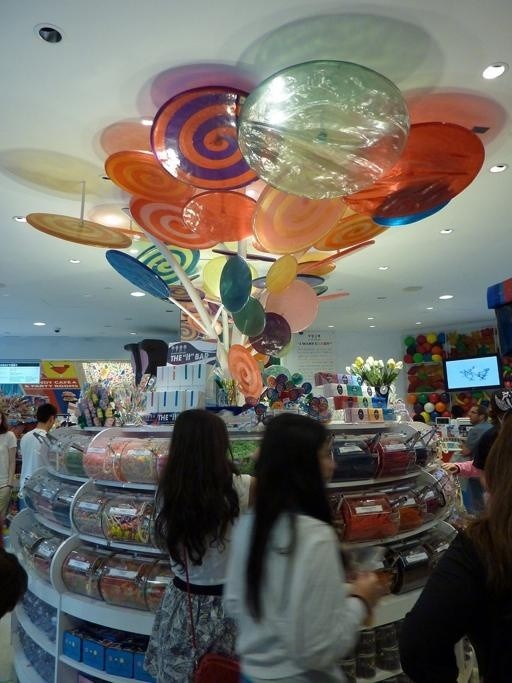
[376,384,390,407]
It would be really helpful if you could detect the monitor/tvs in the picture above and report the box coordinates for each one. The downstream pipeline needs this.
[442,353,504,392]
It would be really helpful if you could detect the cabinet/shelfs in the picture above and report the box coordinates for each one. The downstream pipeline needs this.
[401,322,502,423]
[3,419,480,683]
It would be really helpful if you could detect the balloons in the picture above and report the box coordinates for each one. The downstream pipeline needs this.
[397,327,512,424]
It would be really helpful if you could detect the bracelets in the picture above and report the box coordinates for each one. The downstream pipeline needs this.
[349,590,373,620]
[6,484,14,488]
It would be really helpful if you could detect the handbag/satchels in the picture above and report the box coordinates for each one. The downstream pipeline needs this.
[443,635,481,682]
[195,654,241,682]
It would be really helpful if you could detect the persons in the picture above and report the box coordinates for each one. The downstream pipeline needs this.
[0,410,20,524]
[18,403,59,512]
[221,412,393,683]
[0,543,31,622]
[397,407,512,682]
[139,406,256,683]
[326,370,382,420]
[407,388,512,516]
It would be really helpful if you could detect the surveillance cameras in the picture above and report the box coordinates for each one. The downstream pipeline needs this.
[33,23,64,43]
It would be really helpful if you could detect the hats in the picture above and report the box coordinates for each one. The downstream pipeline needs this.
[491,388,512,412]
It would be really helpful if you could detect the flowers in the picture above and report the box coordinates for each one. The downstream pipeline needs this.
[344,353,403,387]
